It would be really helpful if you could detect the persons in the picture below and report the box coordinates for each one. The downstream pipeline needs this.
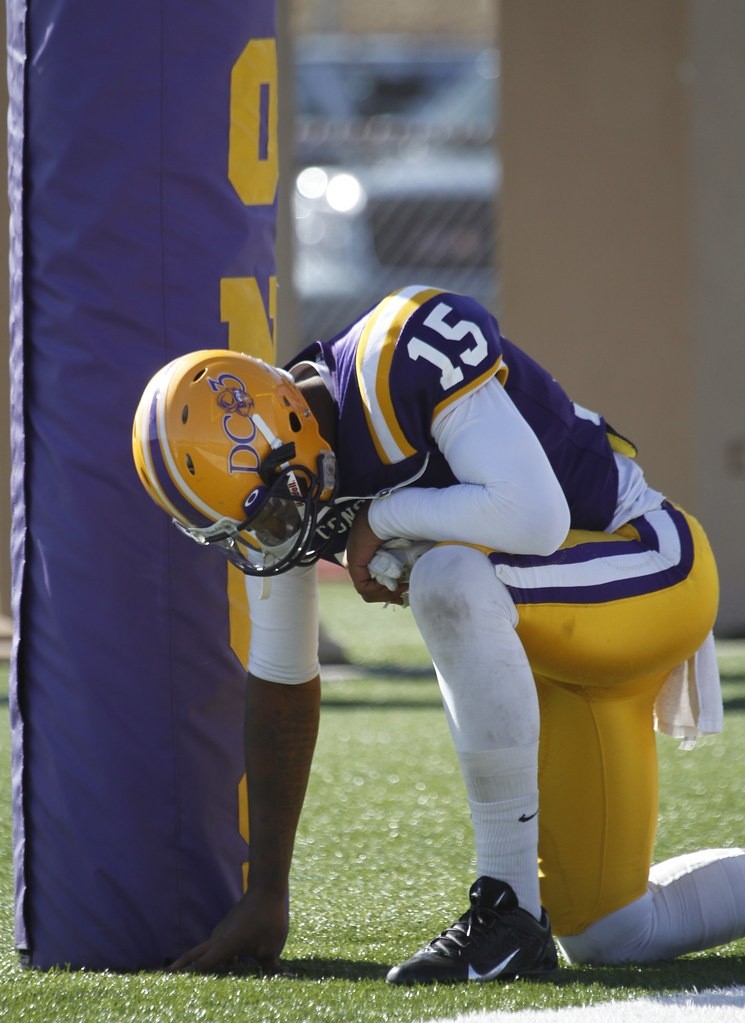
[129,283,745,991]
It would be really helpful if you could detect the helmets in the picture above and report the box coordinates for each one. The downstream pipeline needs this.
[130,348,342,575]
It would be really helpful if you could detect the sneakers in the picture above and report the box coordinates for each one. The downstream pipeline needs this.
[387,878,564,986]
